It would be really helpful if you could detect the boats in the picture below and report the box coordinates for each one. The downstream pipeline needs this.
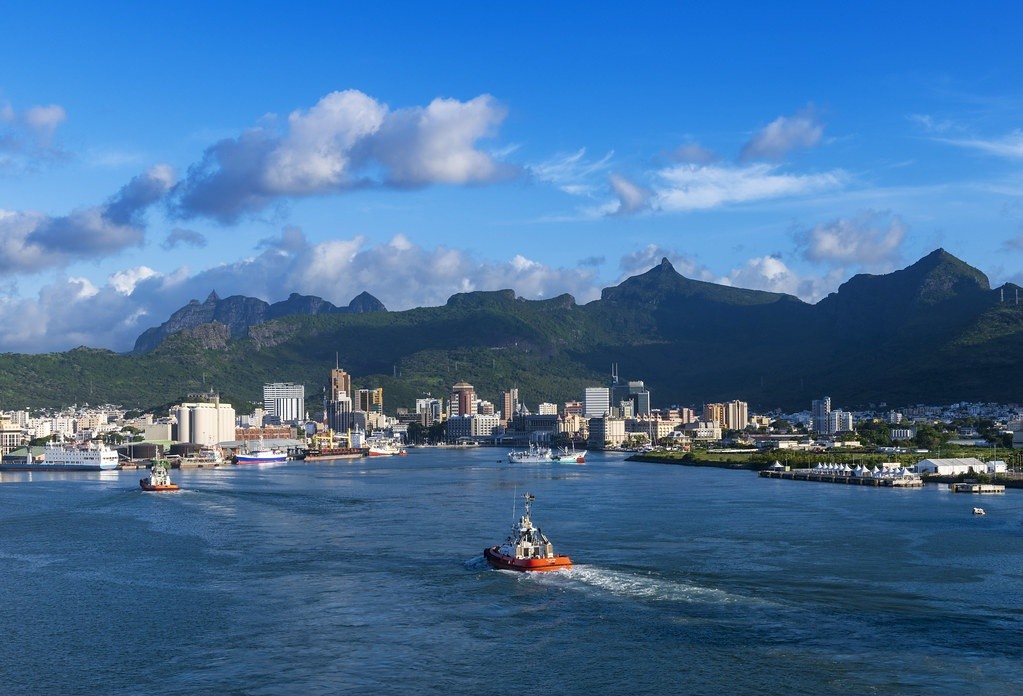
[483,490,573,573]
[235,435,289,464]
[180,446,225,468]
[507,439,587,463]
[368,442,402,459]
[399,449,408,456]
[139,445,180,490]
[299,427,370,462]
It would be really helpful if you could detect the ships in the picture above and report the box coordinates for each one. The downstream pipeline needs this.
[0,439,119,471]
